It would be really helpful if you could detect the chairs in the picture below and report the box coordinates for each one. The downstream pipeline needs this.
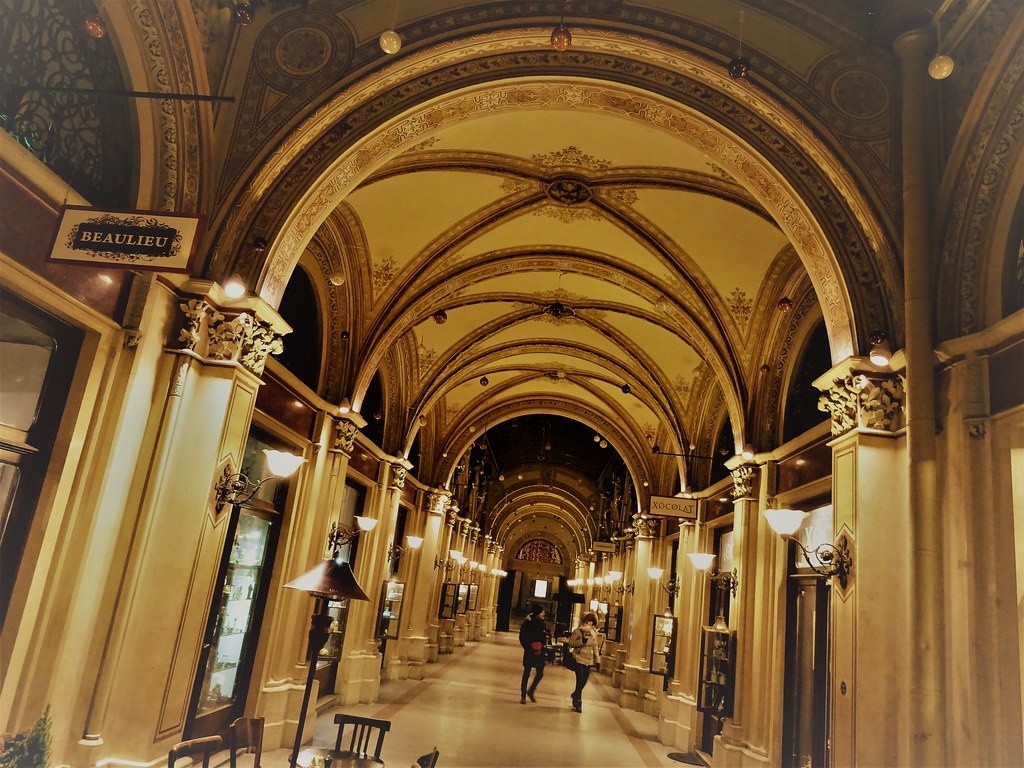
[168,714,439,767]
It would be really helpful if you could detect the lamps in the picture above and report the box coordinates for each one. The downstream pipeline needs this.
[339,397,350,413]
[764,509,853,589]
[328,516,379,551]
[741,443,755,460]
[687,553,738,598]
[565,570,635,594]
[434,549,508,578]
[213,448,307,516]
[869,337,891,367]
[647,567,680,597]
[397,449,404,461]
[274,548,372,767]
[224,272,247,300]
[387,536,424,563]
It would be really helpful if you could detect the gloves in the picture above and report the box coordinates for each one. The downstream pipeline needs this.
[582,637,587,644]
[596,663,599,671]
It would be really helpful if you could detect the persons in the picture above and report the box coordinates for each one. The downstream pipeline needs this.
[570,612,601,713]
[519,605,546,704]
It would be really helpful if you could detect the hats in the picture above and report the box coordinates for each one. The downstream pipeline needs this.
[531,604,544,614]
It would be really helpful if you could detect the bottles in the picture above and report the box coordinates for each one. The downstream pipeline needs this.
[311,754,332,768]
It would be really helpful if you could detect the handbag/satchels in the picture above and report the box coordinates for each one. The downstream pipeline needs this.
[564,652,576,671]
[531,641,541,656]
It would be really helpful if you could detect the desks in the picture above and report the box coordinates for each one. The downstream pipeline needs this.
[287,748,386,767]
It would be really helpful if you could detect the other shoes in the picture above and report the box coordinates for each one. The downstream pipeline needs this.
[576,700,582,712]
[527,690,535,702]
[571,693,575,706]
[521,695,526,704]
